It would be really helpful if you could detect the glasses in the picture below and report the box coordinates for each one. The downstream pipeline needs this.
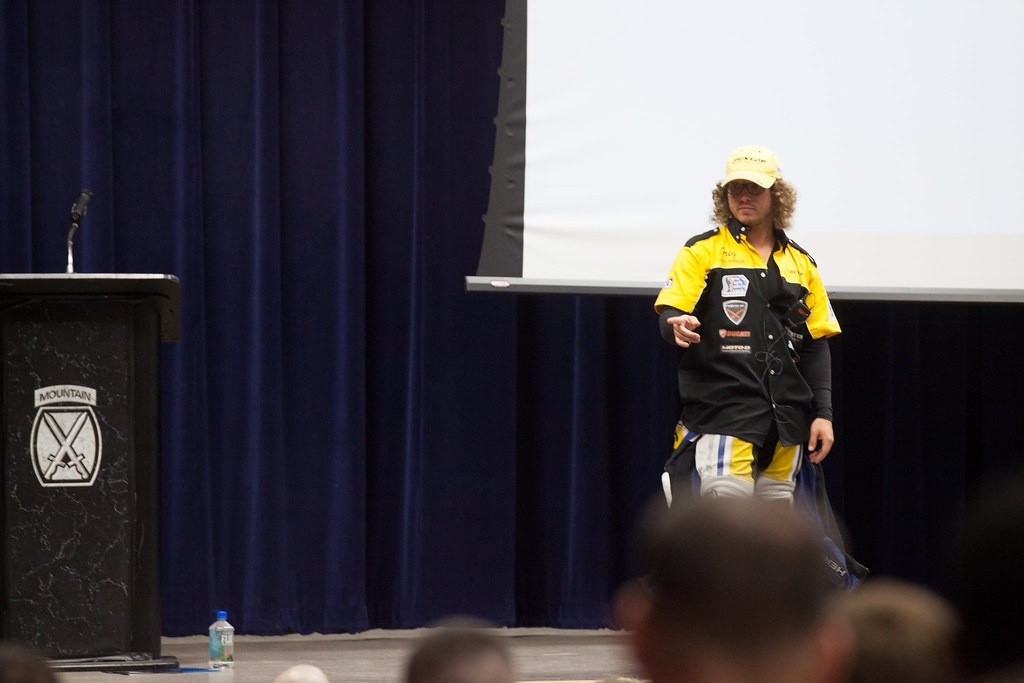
[726,182,765,195]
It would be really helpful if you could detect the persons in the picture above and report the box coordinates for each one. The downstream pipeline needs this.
[654,148,869,600]
[0,644,58,683]
[622,503,1024,683]
[406,630,513,683]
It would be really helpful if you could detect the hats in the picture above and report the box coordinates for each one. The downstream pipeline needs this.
[720,144,779,188]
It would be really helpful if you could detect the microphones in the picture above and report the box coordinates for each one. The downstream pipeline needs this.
[73,190,94,220]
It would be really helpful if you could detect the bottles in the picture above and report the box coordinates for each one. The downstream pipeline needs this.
[209,611,235,670]
